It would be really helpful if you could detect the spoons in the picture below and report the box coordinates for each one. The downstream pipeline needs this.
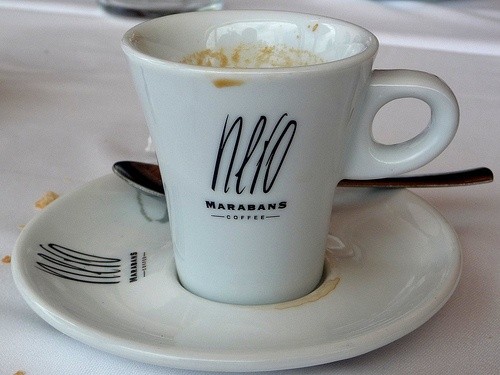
[113,159,495,198]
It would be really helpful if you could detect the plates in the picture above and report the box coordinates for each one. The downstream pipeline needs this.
[12,166,462,374]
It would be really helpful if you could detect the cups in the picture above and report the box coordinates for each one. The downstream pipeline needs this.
[121,11,460,305]
